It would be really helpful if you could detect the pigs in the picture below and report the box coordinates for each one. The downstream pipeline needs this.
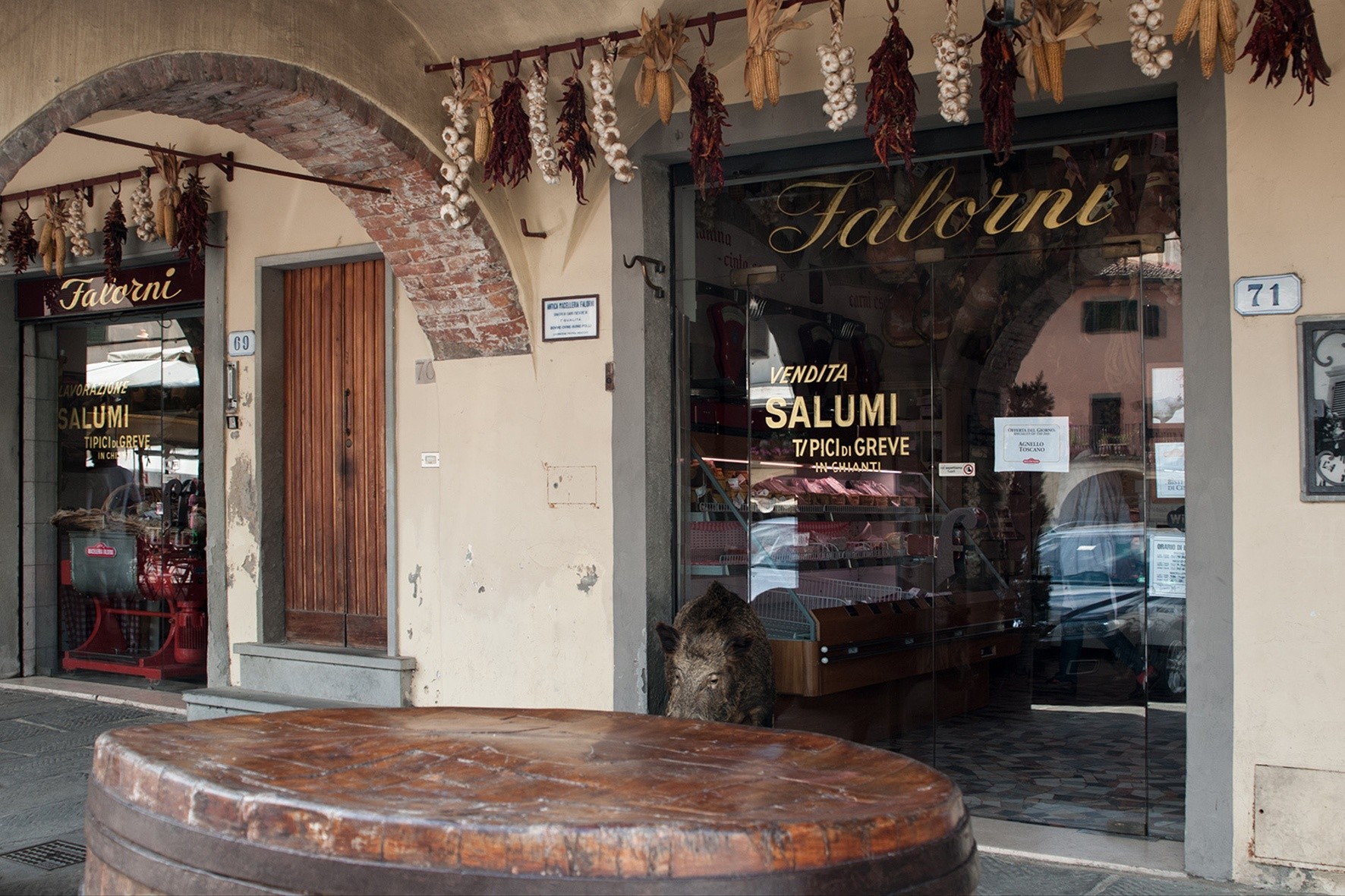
[655,580,776,729]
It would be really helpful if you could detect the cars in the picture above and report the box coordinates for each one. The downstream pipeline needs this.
[1036,520,1186,699]
[750,516,797,602]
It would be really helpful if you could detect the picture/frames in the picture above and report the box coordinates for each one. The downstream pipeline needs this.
[1140,359,1187,603]
[541,292,599,343]
[1294,312,1345,504]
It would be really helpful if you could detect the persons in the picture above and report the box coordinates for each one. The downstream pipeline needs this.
[59,434,142,517]
[1031,424,1161,703]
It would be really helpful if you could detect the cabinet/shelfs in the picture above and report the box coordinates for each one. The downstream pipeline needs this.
[683,459,1038,745]
